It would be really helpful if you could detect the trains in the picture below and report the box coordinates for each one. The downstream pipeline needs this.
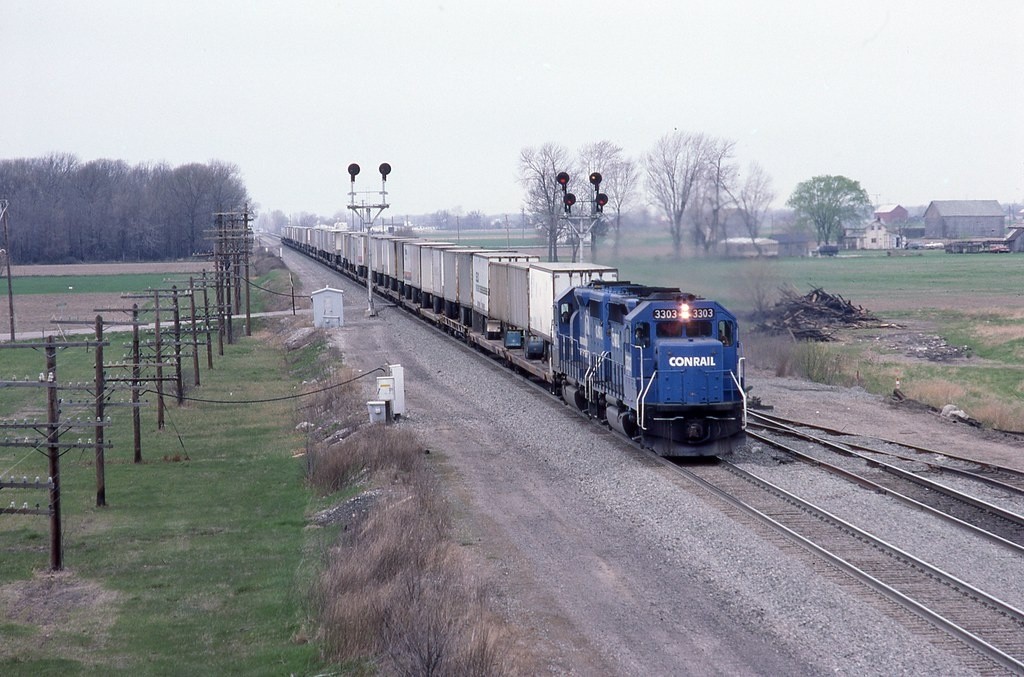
[279,224,747,457]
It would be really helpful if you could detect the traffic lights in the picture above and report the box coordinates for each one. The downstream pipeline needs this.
[557,172,570,184]
[590,172,602,185]
[596,193,608,206]
[563,193,576,206]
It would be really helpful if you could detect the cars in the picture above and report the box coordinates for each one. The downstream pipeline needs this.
[905,242,925,249]
[980,244,1011,253]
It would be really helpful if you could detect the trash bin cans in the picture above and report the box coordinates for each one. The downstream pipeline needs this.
[811,251,818,257]
[366,401,386,424]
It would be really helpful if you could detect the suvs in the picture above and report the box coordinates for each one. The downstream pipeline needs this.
[813,245,838,256]
